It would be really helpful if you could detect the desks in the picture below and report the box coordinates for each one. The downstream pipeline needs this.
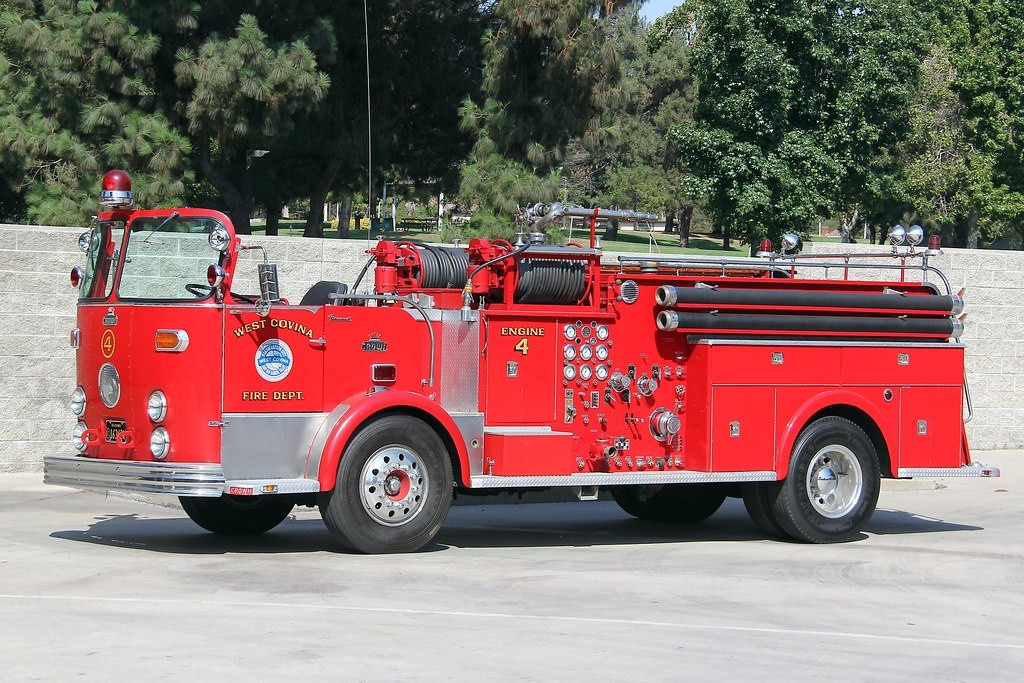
[402,218,432,232]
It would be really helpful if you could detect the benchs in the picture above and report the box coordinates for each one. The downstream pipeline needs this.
[398,223,439,232]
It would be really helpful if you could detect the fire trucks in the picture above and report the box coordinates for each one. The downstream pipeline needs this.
[41,169,999,553]
